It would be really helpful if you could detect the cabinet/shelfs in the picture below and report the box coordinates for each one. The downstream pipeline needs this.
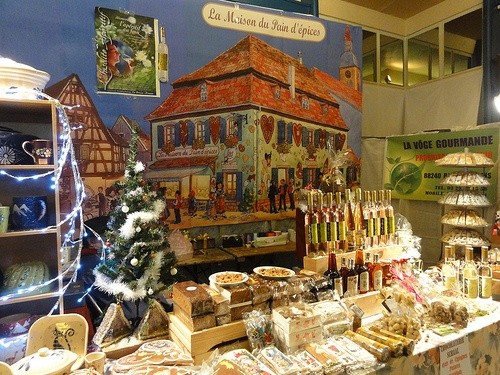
[0,97,63,366]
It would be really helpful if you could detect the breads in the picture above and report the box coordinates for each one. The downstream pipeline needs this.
[92,266,336,348]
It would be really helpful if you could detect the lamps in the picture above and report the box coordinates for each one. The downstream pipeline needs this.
[385,75,392,84]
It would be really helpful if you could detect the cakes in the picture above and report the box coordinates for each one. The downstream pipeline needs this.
[435,152,493,246]
[3,260,51,296]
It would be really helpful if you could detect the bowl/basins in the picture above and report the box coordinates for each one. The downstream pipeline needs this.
[1,259,54,300]
[0,312,88,375]
[0,66,51,99]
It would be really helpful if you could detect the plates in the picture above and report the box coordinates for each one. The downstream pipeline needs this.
[208,272,249,286]
[252,265,295,280]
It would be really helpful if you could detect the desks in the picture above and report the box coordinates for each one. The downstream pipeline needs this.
[104,275,500,375]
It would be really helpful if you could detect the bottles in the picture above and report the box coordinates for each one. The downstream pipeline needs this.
[440,245,493,300]
[308,189,420,299]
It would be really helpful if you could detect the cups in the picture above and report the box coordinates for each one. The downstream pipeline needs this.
[22,138,53,165]
[0,126,23,165]
[84,352,105,375]
[9,196,49,231]
[0,206,9,233]
